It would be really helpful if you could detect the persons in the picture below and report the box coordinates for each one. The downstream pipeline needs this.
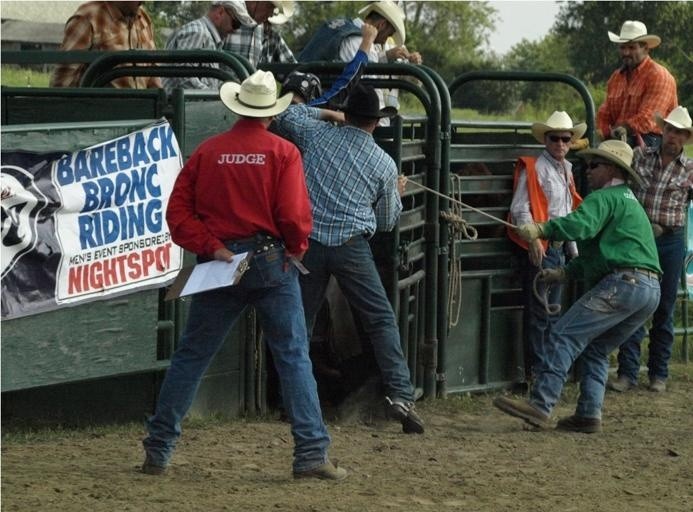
[279,86,426,436]
[607,105,693,393]
[595,20,679,144]
[509,112,586,385]
[492,140,663,433]
[145,72,348,481]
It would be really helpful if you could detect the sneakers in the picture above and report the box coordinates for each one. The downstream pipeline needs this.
[559,414,607,434]
[387,393,427,436]
[649,375,666,393]
[291,458,350,482]
[494,394,549,429]
[611,374,640,392]
[141,456,171,476]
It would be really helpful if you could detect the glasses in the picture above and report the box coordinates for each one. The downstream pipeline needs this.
[547,134,571,143]
[223,6,241,29]
[583,161,616,170]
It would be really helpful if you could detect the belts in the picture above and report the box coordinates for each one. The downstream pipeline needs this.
[617,264,661,282]
[663,225,683,233]
[221,233,279,245]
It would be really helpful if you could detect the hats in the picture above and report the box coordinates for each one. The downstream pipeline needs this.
[218,68,293,119]
[210,1,295,30]
[575,139,646,189]
[363,1,408,47]
[655,104,693,146]
[606,19,661,49]
[530,110,587,145]
[330,85,398,120]
